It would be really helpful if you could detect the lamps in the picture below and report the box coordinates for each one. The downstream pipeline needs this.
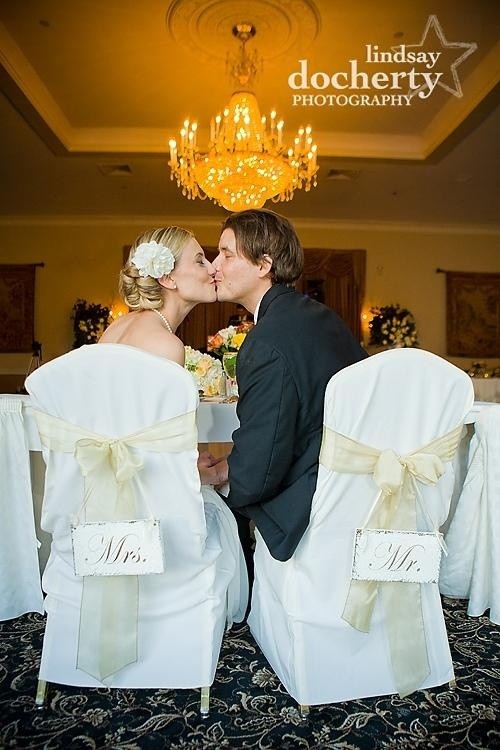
[164,0,320,212]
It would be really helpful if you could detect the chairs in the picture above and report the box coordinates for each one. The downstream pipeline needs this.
[24,343,249,716]
[246,346,475,714]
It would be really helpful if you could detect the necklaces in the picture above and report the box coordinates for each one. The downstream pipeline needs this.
[150,306,172,332]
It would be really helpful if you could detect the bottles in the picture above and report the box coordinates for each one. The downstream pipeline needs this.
[219,371,228,397]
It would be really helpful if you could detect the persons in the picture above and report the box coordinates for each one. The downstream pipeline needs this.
[97,224,218,491]
[202,212,372,635]
[227,303,254,325]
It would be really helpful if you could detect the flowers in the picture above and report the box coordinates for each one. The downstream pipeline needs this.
[207,322,254,356]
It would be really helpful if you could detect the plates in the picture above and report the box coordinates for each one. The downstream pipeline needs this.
[203,398,227,402]
